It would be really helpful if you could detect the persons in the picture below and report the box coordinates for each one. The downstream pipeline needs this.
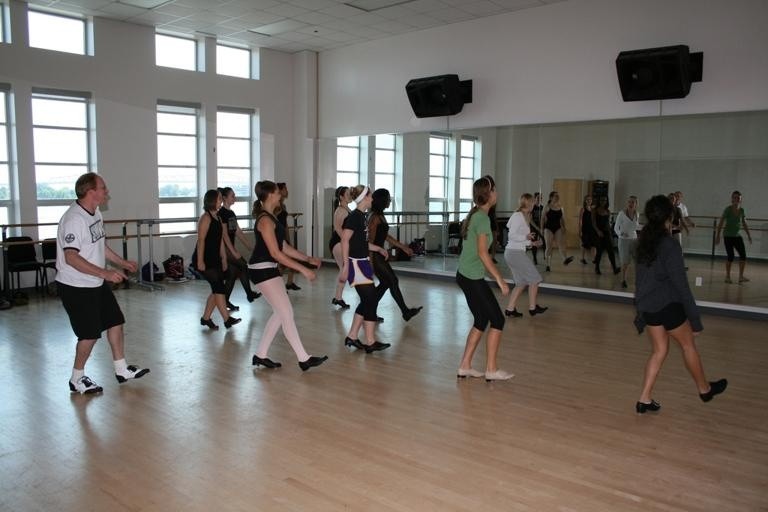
[455,175,516,380]
[532,191,697,288]
[274,182,300,293]
[633,194,727,412]
[246,180,329,373]
[502,193,546,317]
[56,172,151,393]
[715,191,752,284]
[192,187,264,329]
[486,202,498,264]
[329,184,423,356]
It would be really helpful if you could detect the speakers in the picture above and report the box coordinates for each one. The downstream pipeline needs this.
[405,74,464,118]
[616,45,692,102]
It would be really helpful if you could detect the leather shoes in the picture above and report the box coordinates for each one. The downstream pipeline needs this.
[621,280,628,289]
[635,398,662,414]
[456,367,515,383]
[580,259,588,264]
[247,291,263,303]
[698,377,729,403]
[69,375,104,396]
[614,267,623,275]
[504,304,549,318]
[298,354,329,372]
[285,282,302,290]
[546,266,550,272]
[594,268,602,275]
[492,257,498,264]
[200,302,241,330]
[345,336,391,354]
[401,305,424,322]
[252,354,282,369]
[738,278,750,283]
[592,260,596,264]
[376,315,385,322]
[331,297,351,308]
[724,277,733,283]
[115,364,151,384]
[563,255,575,266]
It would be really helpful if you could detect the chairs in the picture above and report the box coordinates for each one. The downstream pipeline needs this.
[2,232,46,306]
[40,239,62,301]
[448,220,463,256]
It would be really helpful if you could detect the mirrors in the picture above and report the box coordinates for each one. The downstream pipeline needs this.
[310,107,767,313]
